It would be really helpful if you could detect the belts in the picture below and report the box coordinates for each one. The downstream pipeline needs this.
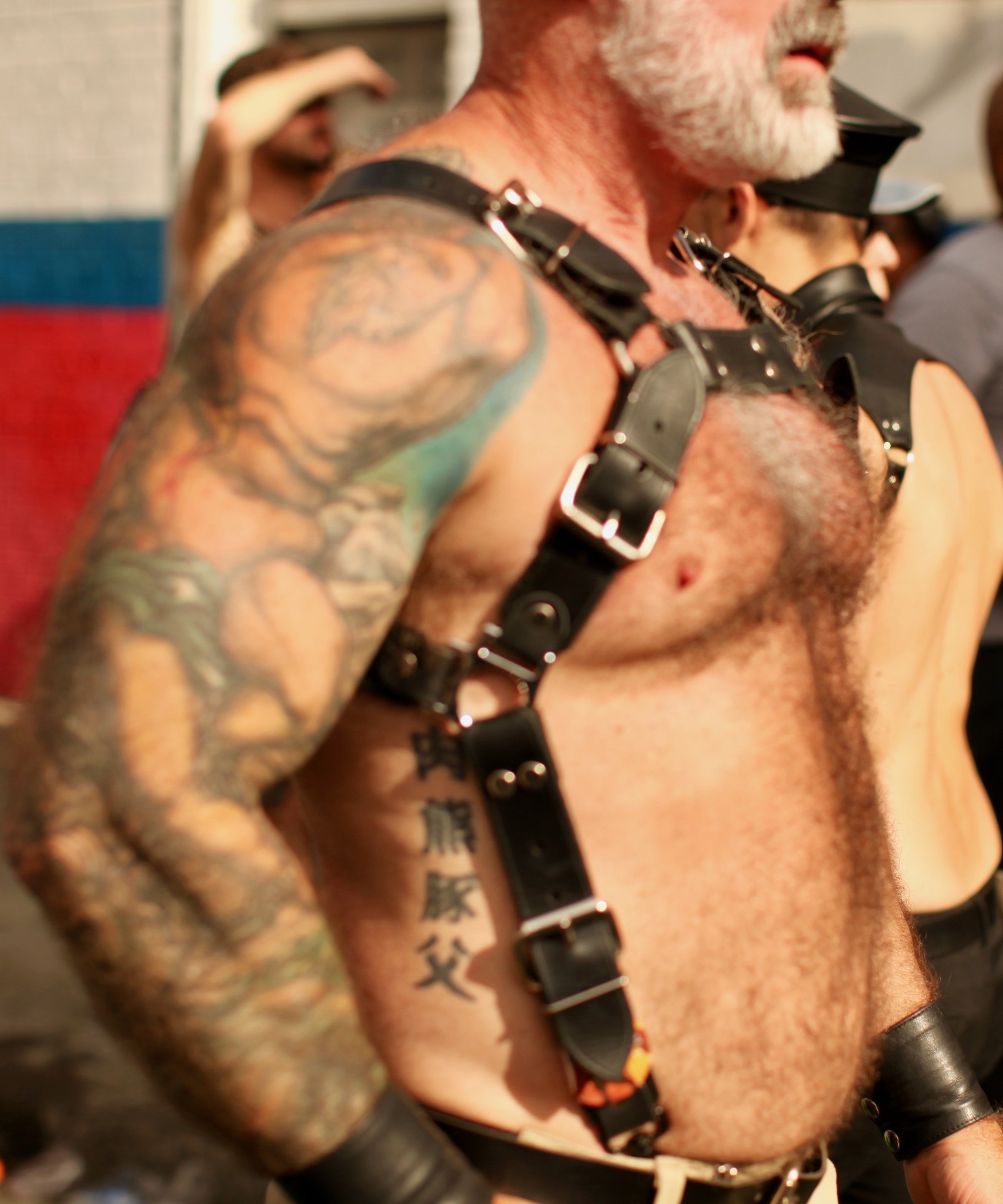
[920,873,1003,960]
[419,1104,827,1204]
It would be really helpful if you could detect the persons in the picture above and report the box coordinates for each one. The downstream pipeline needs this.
[688,73,1003,1203]
[0,2,1003,1204]
[157,41,402,364]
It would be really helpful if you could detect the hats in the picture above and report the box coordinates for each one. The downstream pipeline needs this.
[755,74,923,217]
